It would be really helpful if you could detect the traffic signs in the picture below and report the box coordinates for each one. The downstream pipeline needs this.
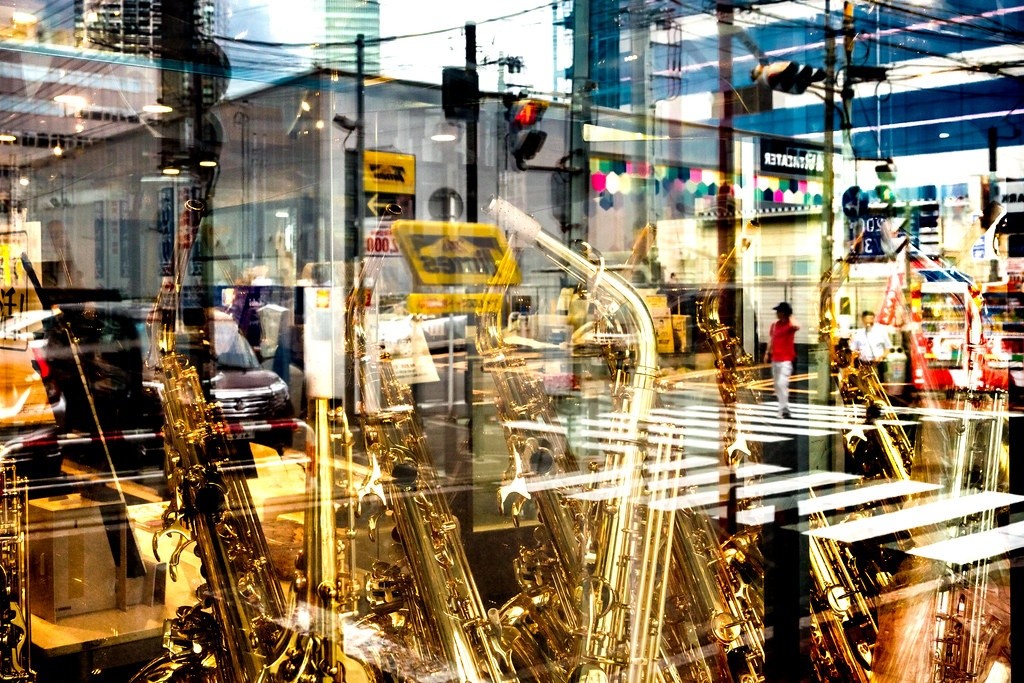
[365,150,416,255]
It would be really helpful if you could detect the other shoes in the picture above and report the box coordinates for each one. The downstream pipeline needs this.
[782,407,791,419]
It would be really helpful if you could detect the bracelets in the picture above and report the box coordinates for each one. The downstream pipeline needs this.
[766,351,770,353]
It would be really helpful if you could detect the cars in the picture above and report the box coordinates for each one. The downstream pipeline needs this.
[377,301,468,353]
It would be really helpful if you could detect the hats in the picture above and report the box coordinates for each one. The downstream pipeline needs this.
[772,301,793,314]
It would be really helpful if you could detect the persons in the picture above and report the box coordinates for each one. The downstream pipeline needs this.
[847,310,892,373]
[234,262,333,285]
[764,302,800,417]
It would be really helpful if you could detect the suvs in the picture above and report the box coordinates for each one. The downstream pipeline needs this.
[44,300,294,462]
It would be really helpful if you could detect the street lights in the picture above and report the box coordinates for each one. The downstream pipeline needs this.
[330,114,365,424]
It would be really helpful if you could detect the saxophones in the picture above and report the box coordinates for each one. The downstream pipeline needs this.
[1,185,1021,681]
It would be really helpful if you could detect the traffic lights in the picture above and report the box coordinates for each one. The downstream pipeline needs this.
[442,66,480,122]
[508,96,550,171]
[752,59,826,95]
